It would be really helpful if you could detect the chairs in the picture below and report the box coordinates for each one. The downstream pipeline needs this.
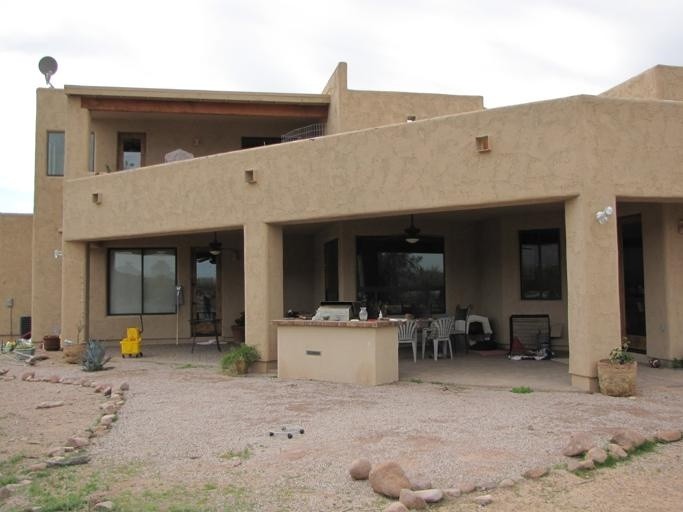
[397,320,418,364]
[421,317,453,360]
[448,304,472,353]
[189,311,222,354]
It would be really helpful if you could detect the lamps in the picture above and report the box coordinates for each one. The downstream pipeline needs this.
[595,206,613,224]
[405,214,420,244]
[207,233,222,255]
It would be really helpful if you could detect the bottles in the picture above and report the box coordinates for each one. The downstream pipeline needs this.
[358,307,368,320]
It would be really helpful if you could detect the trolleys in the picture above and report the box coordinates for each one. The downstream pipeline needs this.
[120,315,143,358]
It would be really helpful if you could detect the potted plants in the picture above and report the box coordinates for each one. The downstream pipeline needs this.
[62,324,89,364]
[220,344,259,374]
[15,339,36,360]
[231,312,246,341]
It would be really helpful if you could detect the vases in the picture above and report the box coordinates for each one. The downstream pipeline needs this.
[43,335,60,351]
[597,336,637,396]
